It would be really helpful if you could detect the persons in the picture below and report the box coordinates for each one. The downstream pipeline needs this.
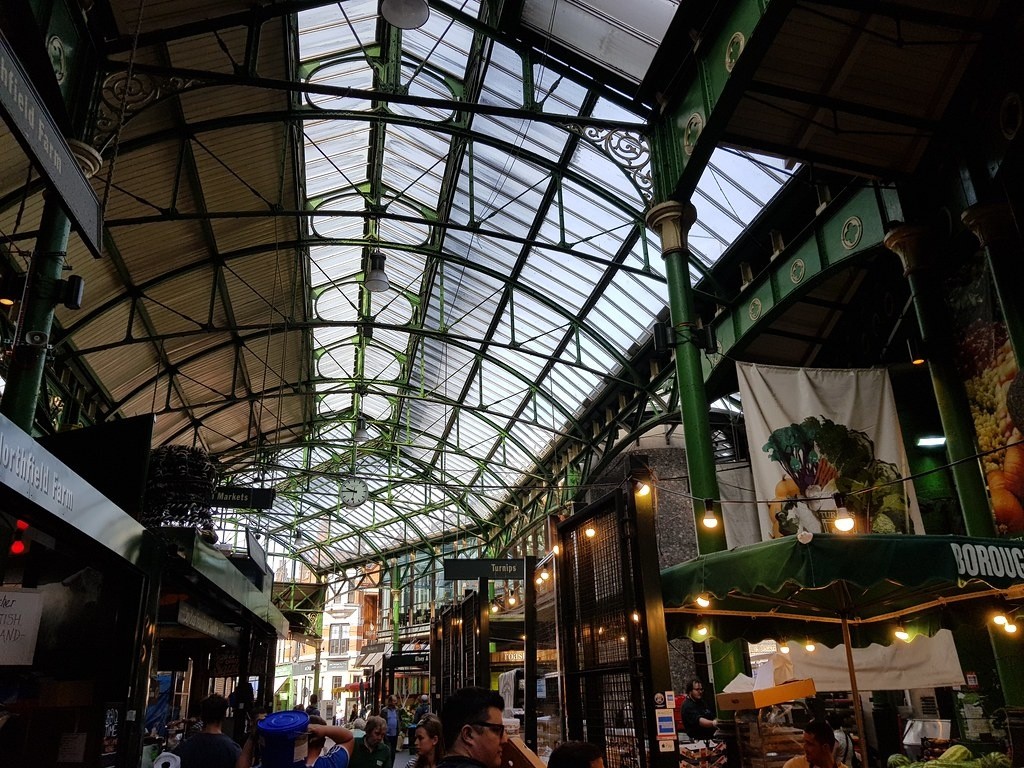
[173,685,605,768]
[307,694,320,716]
[679,680,719,740]
[827,713,854,768]
[380,695,400,768]
[782,719,848,768]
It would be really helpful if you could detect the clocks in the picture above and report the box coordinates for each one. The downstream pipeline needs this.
[339,477,368,507]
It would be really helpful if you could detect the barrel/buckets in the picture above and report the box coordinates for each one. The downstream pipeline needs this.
[258,711,310,768]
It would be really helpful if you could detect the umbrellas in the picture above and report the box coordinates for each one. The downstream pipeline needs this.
[659,531,1024,768]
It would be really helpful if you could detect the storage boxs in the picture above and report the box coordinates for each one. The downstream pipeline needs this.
[964,701,984,718]
[967,719,989,740]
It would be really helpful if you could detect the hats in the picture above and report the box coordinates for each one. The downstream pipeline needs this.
[421,694,429,700]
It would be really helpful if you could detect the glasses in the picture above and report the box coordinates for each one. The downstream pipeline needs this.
[693,689,703,692]
[473,720,505,738]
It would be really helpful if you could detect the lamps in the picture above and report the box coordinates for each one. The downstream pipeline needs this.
[381,0,430,30]
[364,217,390,292]
